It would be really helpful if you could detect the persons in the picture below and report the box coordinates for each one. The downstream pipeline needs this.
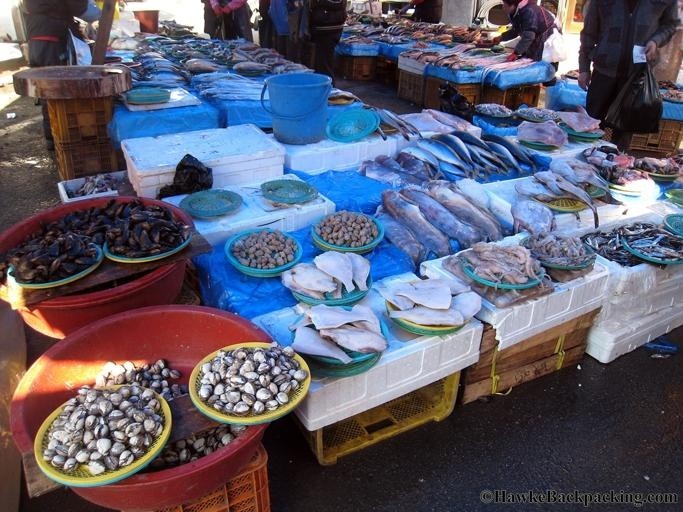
[19,1,102,152]
[200,0,347,87]
[398,0,443,24]
[491,1,567,87]
[577,2,681,155]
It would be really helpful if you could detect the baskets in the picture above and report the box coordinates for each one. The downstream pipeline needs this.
[46,98,122,178]
[330,54,541,113]
[150,437,273,511]
[600,120,683,160]
[293,368,464,469]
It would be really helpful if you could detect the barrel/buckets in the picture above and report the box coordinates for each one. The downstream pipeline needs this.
[132,10,160,34]
[260,72,333,144]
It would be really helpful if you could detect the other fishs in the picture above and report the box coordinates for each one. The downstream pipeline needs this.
[357,104,683,286]
